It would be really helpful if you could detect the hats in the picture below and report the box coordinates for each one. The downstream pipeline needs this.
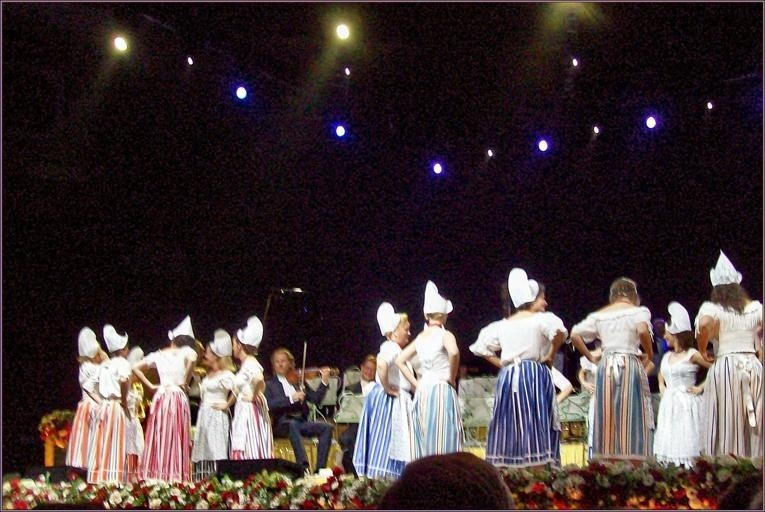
[167,314,195,340]
[423,279,454,319]
[506,267,540,308]
[237,315,264,348]
[376,301,401,336]
[708,248,742,286]
[209,328,233,357]
[77,326,98,359]
[102,323,129,353]
[664,301,692,335]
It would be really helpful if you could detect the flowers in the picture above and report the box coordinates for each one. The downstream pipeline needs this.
[37,409,75,448]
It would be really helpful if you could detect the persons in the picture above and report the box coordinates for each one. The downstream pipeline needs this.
[132,315,203,483]
[264,348,333,475]
[548,366,574,470]
[230,316,274,459]
[570,278,654,468]
[353,302,412,480]
[578,345,655,464]
[694,252,764,476]
[395,281,462,462]
[66,325,152,483]
[341,355,375,473]
[658,301,712,465]
[469,269,568,469]
[191,330,237,462]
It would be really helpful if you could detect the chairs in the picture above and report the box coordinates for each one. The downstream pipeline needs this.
[268,418,347,471]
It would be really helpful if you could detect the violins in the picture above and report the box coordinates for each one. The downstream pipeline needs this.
[287,366,340,383]
